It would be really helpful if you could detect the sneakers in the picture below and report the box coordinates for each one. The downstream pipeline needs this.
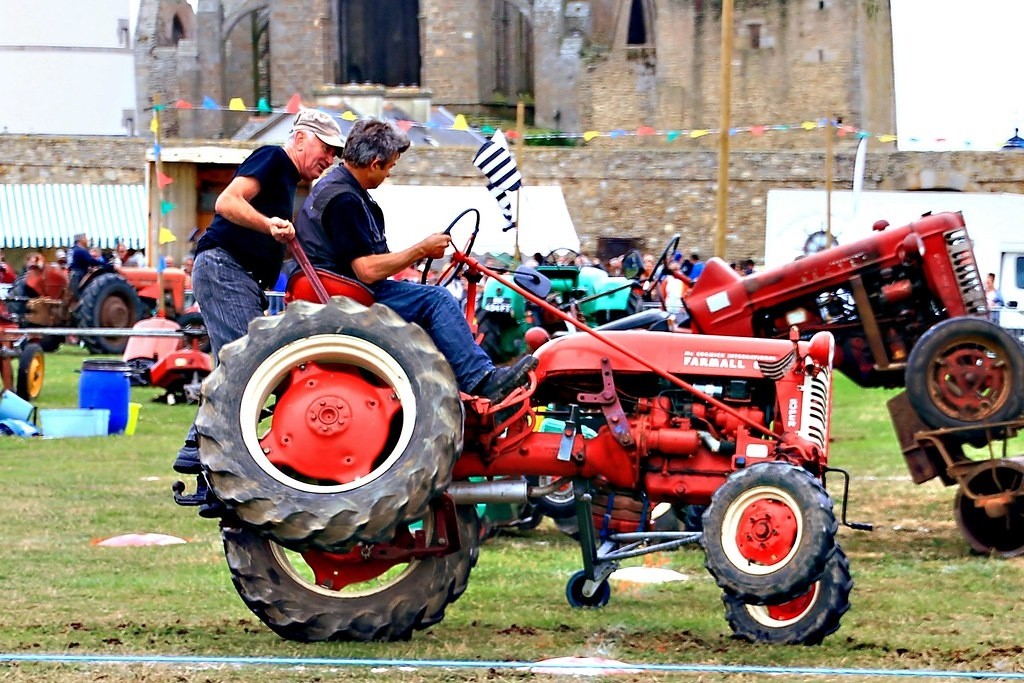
[476,354,539,405]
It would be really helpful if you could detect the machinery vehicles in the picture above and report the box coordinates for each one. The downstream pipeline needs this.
[0,209,1024,648]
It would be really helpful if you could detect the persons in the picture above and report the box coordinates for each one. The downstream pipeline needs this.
[0,233,195,309]
[985,272,1005,324]
[173,109,346,519]
[417,247,759,327]
[294,119,539,407]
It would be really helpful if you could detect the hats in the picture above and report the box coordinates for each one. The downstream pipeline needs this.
[293,109,344,158]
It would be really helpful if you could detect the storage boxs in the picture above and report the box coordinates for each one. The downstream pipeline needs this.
[39,408,111,439]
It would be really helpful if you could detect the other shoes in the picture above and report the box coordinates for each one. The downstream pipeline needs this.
[173,447,203,474]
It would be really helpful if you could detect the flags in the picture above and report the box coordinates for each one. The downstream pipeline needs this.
[471,140,522,192]
[486,182,516,232]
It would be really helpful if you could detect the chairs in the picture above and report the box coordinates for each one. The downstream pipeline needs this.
[283,261,376,308]
[43,264,83,298]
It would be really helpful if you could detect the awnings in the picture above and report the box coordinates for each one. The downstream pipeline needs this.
[365,184,580,257]
[0,184,147,250]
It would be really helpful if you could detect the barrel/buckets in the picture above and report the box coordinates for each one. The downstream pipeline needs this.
[78,358,132,436]
[0,389,35,424]
[124,402,142,435]
